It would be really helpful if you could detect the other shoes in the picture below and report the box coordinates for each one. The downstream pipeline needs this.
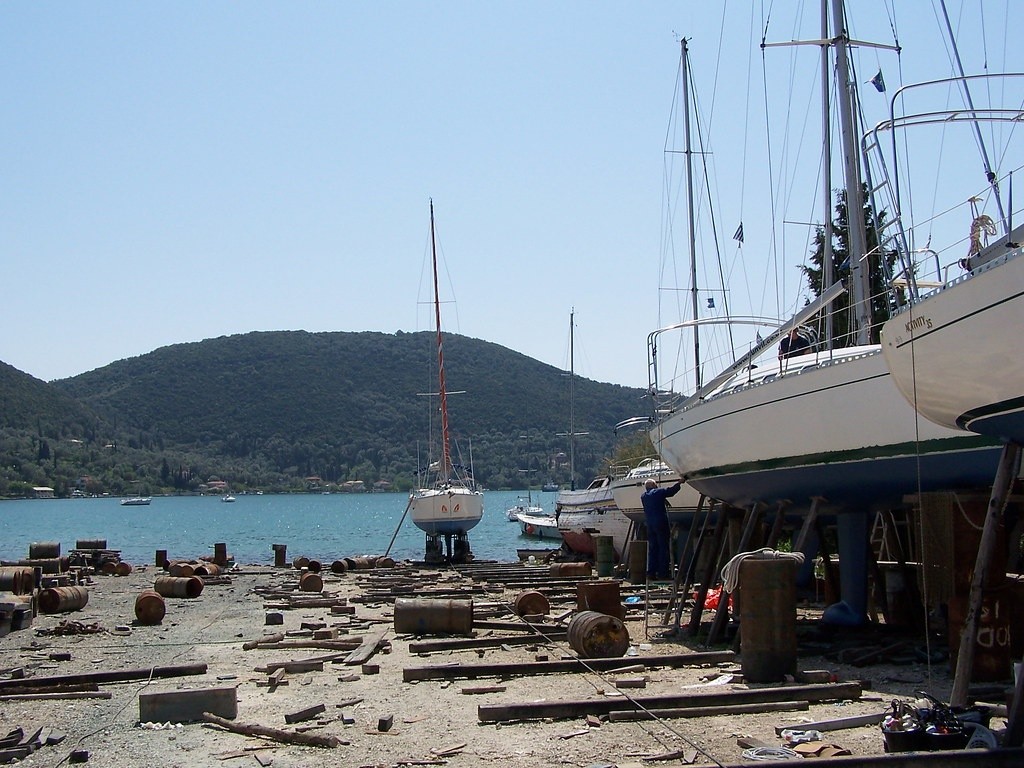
[659,573,671,580]
[648,575,658,580]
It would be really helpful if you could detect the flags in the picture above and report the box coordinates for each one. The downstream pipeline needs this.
[864,68,886,92]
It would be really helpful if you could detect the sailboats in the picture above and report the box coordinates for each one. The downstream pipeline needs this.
[408,198,484,535]
[506,424,544,523]
[553,0,1024,574]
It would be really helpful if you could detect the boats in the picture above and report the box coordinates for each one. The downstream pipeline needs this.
[220,495,236,502]
[120,497,152,505]
[516,511,564,540]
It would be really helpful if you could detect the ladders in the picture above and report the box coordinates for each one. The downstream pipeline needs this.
[869,509,903,561]
[645,524,681,641]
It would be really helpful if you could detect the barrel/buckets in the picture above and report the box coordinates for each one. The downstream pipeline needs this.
[597,534,614,577]
[577,580,620,614]
[570,610,630,659]
[0,540,132,613]
[294,557,309,569]
[631,541,647,584]
[694,536,716,580]
[136,590,166,625]
[515,592,549,616]
[153,543,226,576]
[331,555,395,573]
[300,572,323,592]
[549,562,592,576]
[156,577,202,598]
[736,558,797,682]
[395,599,474,634]
[309,560,322,573]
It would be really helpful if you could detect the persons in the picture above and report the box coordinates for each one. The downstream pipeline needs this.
[778,326,812,360]
[641,479,687,581]
